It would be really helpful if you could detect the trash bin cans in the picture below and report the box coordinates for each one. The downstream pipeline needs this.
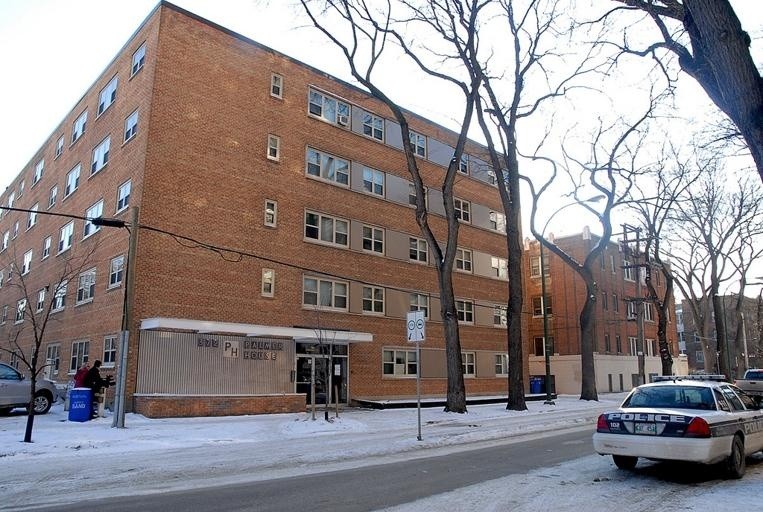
[69,388,93,422]
[531,379,542,394]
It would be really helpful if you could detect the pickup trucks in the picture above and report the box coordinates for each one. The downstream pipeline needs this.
[732,368,762,401]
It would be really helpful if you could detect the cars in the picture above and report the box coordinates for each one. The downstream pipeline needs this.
[591,374,762,479]
[0,361,58,415]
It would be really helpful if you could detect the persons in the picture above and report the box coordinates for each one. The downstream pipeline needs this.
[74,359,109,420]
